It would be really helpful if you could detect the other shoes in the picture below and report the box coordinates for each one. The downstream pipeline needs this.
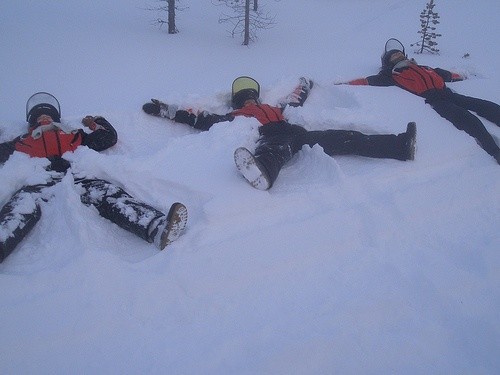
[398,121,417,161]
[150,202,188,250]
[233,147,271,191]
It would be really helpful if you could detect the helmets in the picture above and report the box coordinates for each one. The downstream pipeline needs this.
[381,37,408,71]
[25,91,61,129]
[231,76,260,103]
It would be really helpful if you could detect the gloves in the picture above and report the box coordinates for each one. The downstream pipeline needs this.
[143,99,178,119]
[81,116,94,126]
[298,77,313,90]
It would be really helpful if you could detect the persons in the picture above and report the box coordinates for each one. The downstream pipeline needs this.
[333,38,500,166]
[142,76,417,191]
[0,92,189,263]
